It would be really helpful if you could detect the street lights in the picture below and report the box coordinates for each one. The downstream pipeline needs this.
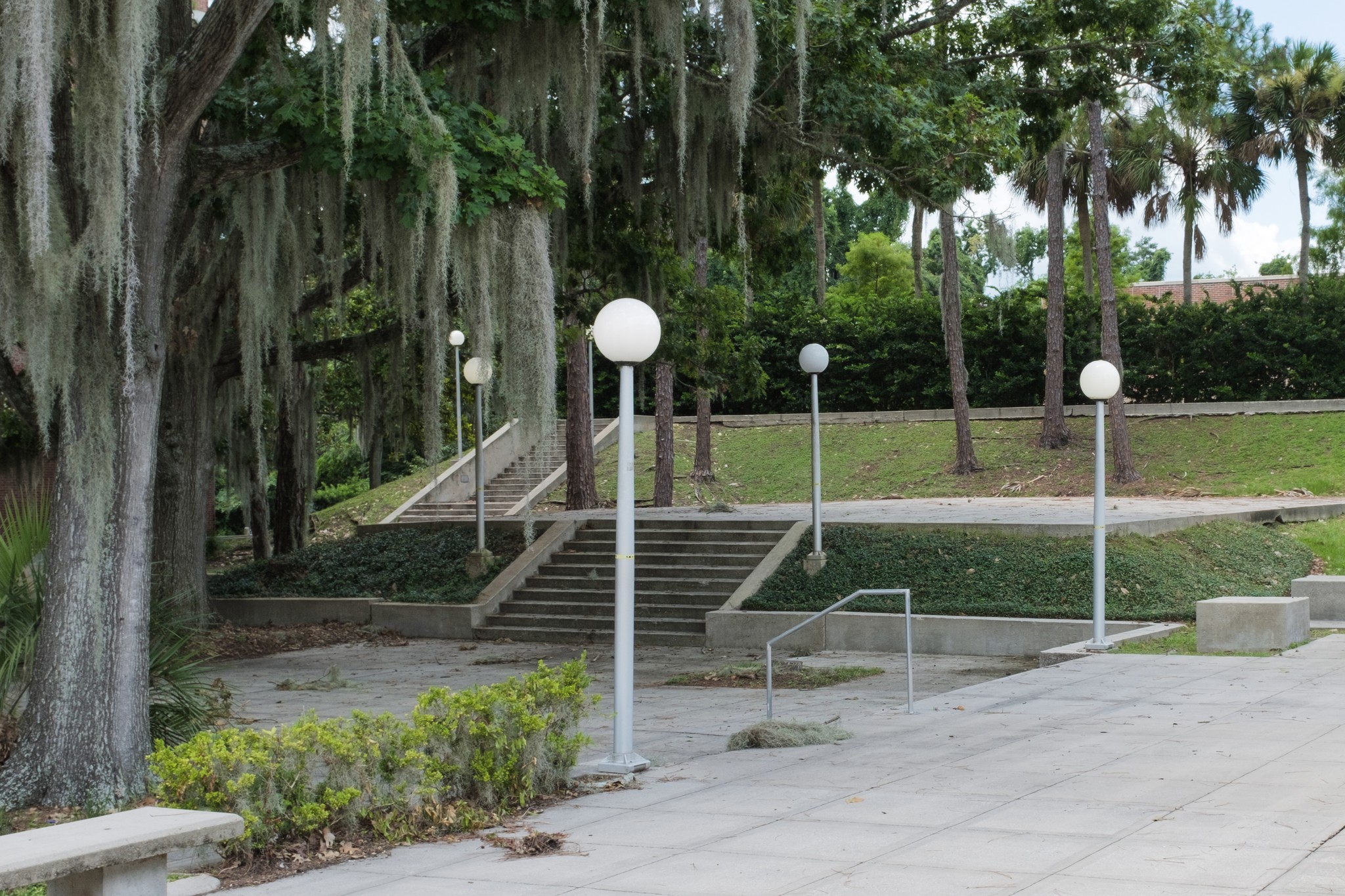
[1079,359,1121,651]
[591,295,663,775]
[799,343,829,560]
[463,357,493,557]
[448,330,465,460]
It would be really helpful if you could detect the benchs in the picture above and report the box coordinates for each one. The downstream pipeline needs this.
[0,804,247,896]
